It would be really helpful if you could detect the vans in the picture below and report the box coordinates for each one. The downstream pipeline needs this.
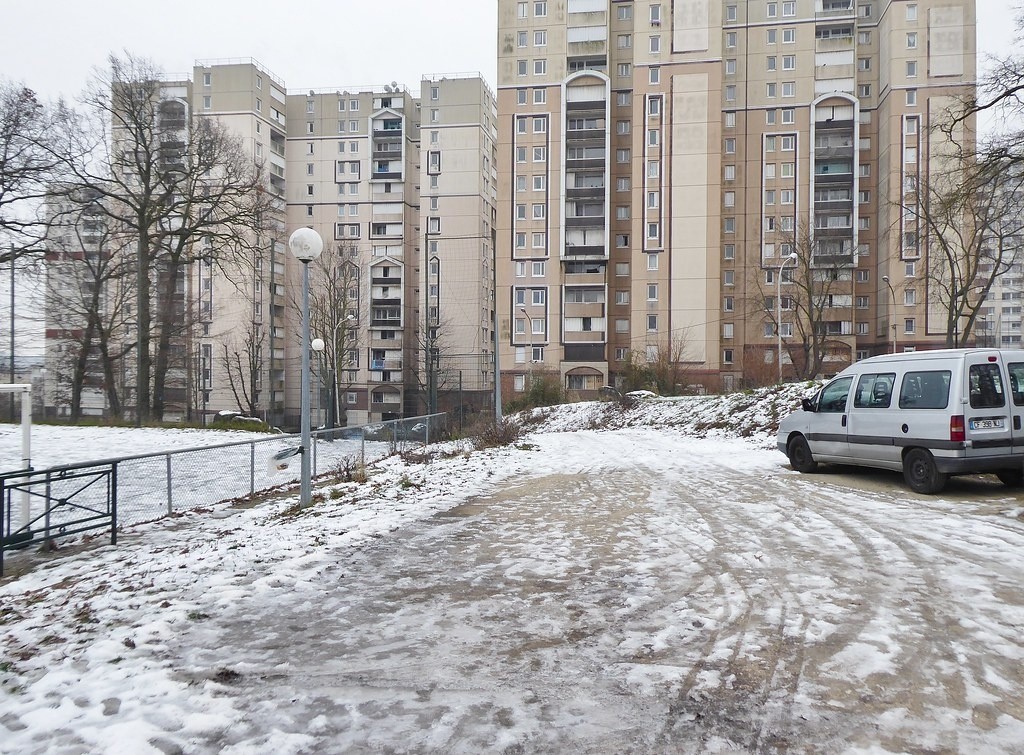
[775,347,1024,496]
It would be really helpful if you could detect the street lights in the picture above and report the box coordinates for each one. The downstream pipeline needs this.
[333,314,355,426]
[288,228,324,510]
[882,275,897,353]
[311,339,325,428]
[779,252,798,385]
[521,308,533,390]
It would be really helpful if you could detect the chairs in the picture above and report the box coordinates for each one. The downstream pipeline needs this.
[854,373,1019,407]
